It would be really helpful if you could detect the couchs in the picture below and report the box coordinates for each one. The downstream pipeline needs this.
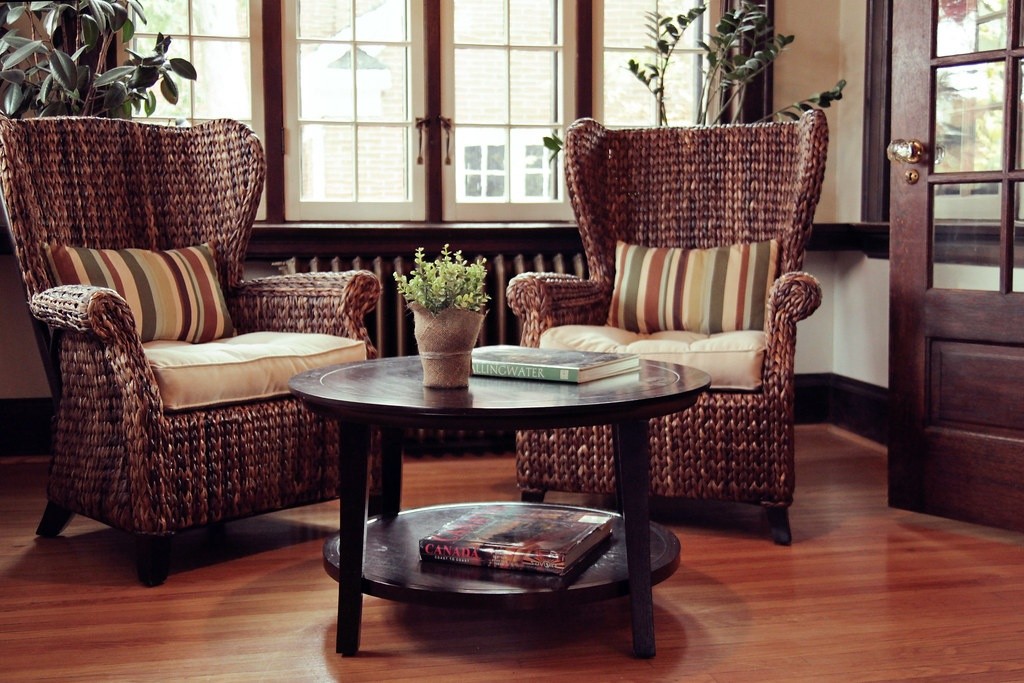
[0,114,384,586]
[505,109,829,546]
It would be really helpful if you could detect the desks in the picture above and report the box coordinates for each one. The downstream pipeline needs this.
[289,347,710,658]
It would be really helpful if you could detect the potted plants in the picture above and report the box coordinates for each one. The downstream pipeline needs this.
[393,242,492,389]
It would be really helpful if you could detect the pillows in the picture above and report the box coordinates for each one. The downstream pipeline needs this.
[605,238,780,331]
[40,239,236,344]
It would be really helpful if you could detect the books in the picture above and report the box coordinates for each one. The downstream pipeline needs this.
[418,505,614,577]
[470,345,642,383]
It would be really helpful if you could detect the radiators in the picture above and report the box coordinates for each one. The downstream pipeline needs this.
[272,250,591,458]
